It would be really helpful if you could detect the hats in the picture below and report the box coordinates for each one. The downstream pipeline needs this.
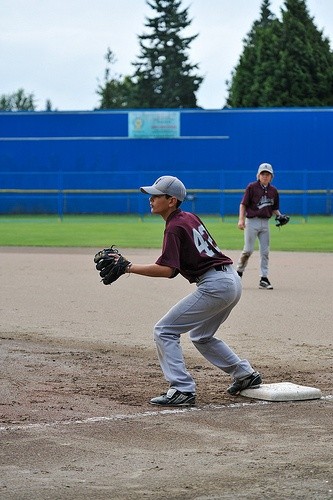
[258,163,273,174]
[140,176,187,203]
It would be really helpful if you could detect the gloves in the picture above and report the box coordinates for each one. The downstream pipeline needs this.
[275,215,289,226]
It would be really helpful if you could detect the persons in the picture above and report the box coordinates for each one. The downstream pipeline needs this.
[235,164,290,290]
[95,176,263,406]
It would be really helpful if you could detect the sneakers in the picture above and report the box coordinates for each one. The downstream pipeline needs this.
[258,277,274,289]
[227,372,262,396]
[150,389,196,406]
